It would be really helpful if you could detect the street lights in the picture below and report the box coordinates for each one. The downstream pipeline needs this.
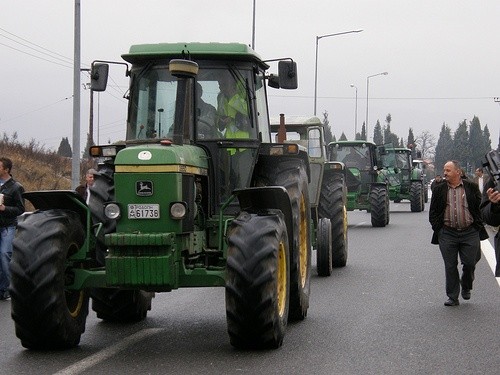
[365,72,390,142]
[313,29,364,118]
[350,83,358,139]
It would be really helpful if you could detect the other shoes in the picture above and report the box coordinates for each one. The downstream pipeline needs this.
[0,292,11,301]
[462,286,471,299]
[444,296,459,306]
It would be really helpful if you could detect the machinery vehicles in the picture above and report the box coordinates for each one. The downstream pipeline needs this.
[10,41,315,352]
[269,116,349,279]
[380,145,428,213]
[327,140,389,228]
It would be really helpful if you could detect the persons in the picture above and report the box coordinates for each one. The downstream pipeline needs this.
[0,157,25,301]
[75,168,97,206]
[479,149,500,277]
[390,155,403,168]
[218,80,249,154]
[429,160,489,305]
[342,147,362,162]
[167,82,216,138]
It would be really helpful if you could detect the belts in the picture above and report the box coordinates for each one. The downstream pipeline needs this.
[444,225,473,233]
